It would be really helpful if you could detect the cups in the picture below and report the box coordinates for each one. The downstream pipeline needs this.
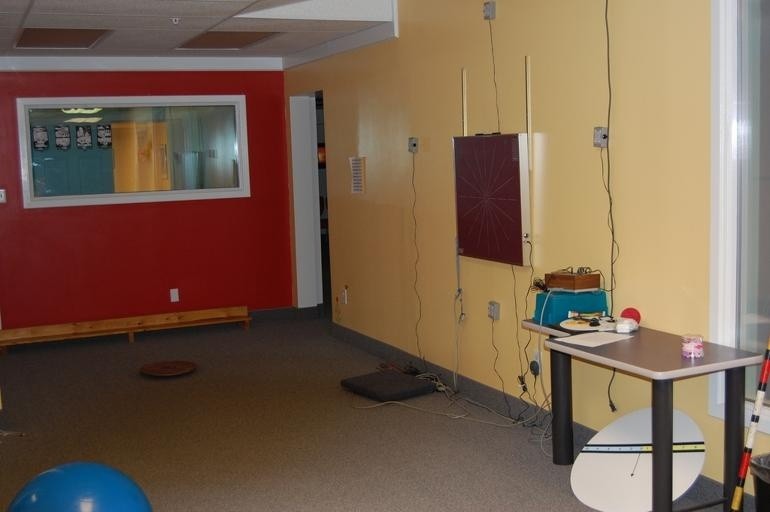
[680,334,704,360]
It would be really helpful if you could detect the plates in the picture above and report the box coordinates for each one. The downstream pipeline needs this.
[558,316,609,332]
[139,359,197,377]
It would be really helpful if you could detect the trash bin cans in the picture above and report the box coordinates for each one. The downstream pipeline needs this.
[750,453,770,512]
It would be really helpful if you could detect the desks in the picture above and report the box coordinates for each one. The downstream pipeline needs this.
[520,316,765,511]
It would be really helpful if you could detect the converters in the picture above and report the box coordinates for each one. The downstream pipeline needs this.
[530,361,539,376]
